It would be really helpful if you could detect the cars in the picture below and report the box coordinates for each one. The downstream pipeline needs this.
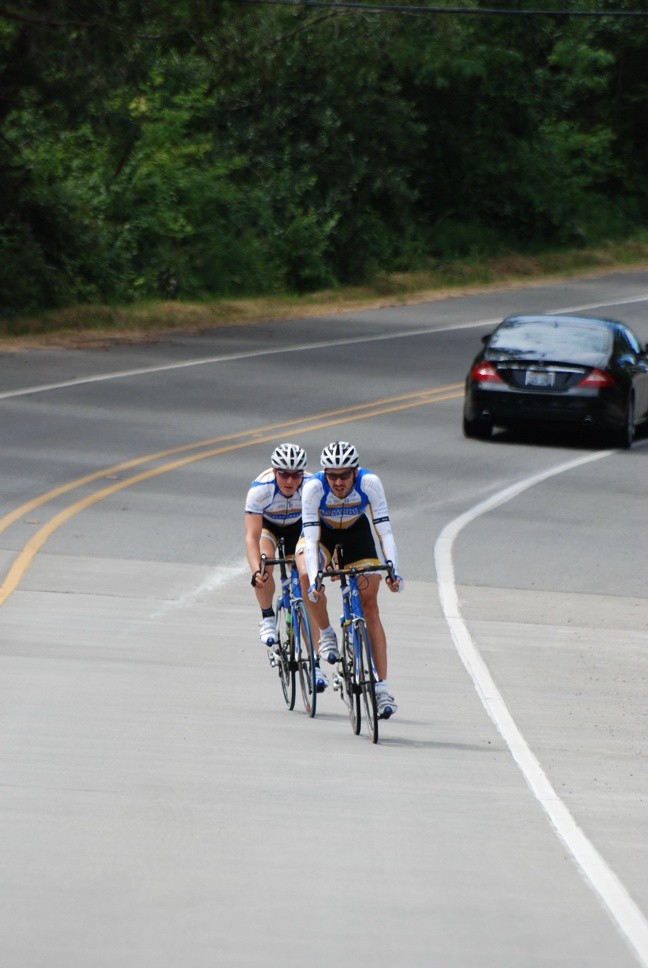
[463,312,648,449]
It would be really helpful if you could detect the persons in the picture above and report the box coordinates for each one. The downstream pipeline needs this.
[244,443,328,692]
[295,441,404,717]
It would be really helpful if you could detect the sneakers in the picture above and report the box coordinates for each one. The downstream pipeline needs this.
[260,616,278,646]
[315,667,328,689]
[318,632,340,664]
[374,683,398,716]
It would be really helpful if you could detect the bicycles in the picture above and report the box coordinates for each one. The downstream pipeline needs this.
[314,538,398,745]
[258,533,327,717]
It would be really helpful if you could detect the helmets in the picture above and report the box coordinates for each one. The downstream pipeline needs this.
[321,441,359,468]
[271,443,308,470]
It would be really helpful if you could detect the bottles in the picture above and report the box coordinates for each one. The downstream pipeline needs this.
[349,628,353,653]
[286,606,291,625]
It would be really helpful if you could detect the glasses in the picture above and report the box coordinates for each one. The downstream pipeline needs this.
[324,469,353,481]
[276,470,303,480]
